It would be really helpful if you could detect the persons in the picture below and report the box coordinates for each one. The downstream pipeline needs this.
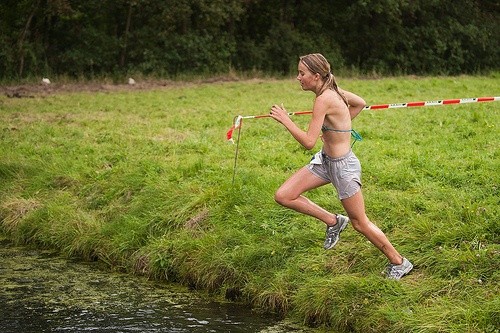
[270,53,414,278]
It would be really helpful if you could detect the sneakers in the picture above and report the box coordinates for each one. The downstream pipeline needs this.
[386,256,413,279]
[323,213,348,251]
